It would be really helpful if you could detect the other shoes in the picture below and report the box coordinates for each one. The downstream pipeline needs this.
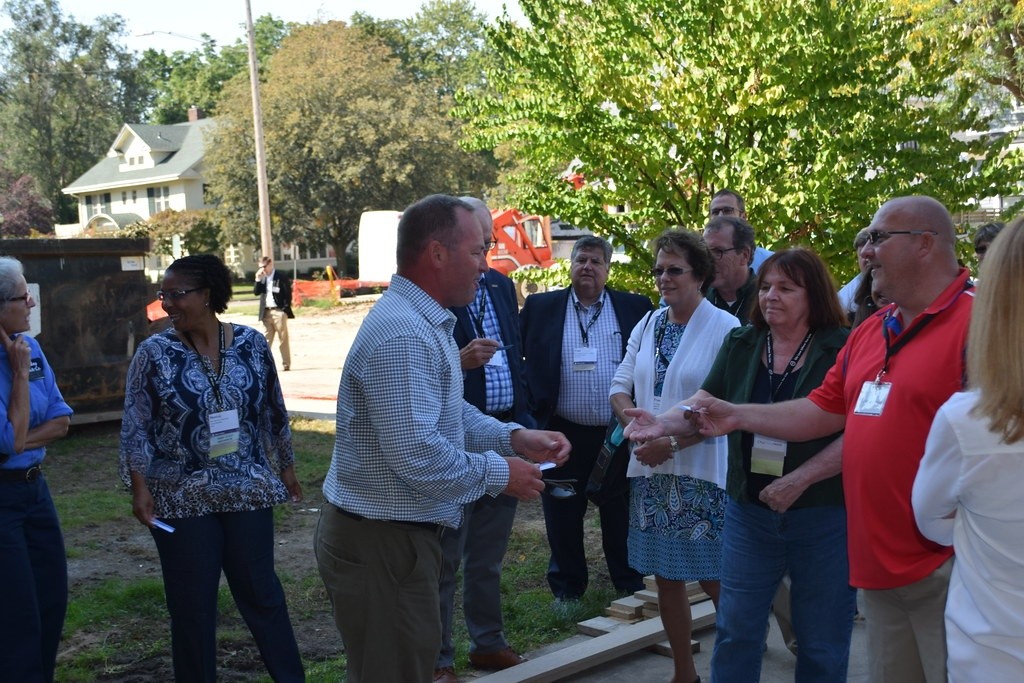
[285,366,288,371]
[555,598,575,627]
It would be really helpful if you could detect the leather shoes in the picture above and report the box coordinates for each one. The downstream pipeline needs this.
[433,666,458,683]
[469,645,528,672]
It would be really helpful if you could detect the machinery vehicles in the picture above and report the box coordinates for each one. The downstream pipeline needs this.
[292,204,555,308]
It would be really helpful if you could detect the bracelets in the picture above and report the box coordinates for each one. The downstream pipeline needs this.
[669,435,679,453]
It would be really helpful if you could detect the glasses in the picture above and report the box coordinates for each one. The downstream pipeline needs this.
[652,267,697,277]
[710,245,742,259]
[868,230,940,246]
[973,244,987,254]
[157,286,207,301]
[711,205,744,216]
[485,231,499,250]
[7,288,30,304]
[259,264,269,269]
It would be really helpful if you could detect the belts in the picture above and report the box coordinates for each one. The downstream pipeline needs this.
[0,464,42,481]
[265,307,279,309]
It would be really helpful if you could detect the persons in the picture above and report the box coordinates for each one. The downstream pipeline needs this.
[609,189,1024,683]
[0,255,73,683]
[515,235,654,603]
[253,255,296,372]
[436,198,542,683]
[113,255,307,683]
[318,196,569,683]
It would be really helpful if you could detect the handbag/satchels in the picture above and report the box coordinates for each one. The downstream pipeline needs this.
[585,401,637,507]
